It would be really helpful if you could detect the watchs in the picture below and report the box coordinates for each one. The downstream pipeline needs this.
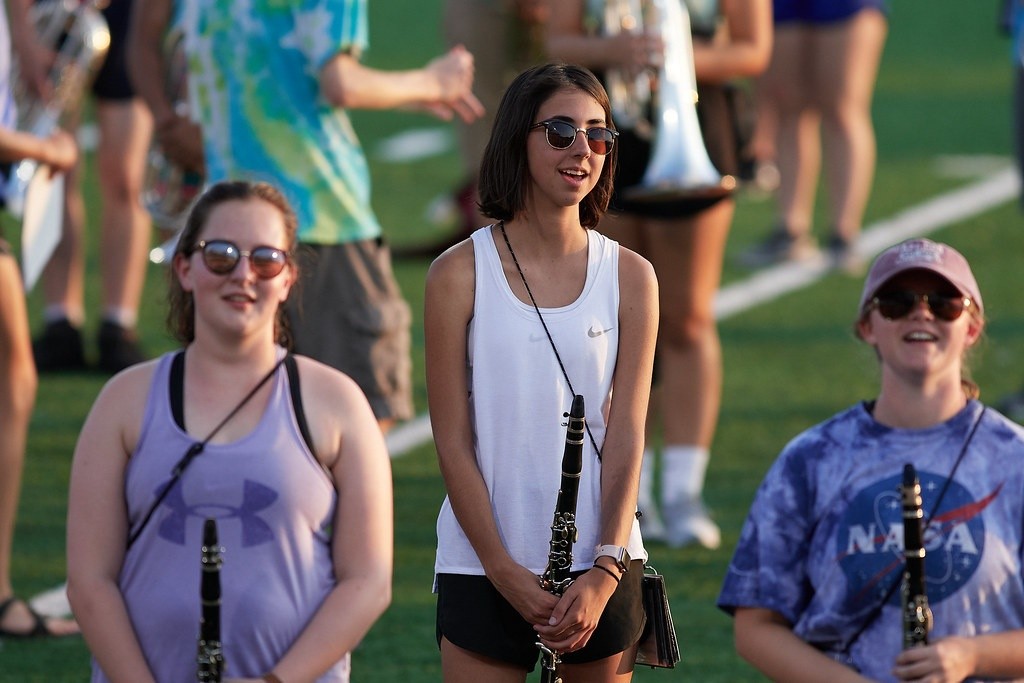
[593,543,632,573]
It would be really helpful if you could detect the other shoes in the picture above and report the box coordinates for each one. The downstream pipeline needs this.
[741,231,820,271]
[1,593,68,639]
[660,494,723,551]
[639,506,667,545]
[31,317,85,373]
[95,321,146,371]
[827,231,869,279]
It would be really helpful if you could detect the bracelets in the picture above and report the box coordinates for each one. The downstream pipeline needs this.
[593,564,620,586]
[263,672,282,683]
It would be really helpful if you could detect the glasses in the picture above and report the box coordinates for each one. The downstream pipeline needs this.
[190,240,291,279]
[528,119,620,157]
[860,285,977,323]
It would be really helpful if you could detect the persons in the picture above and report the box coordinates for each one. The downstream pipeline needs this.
[423,62,660,683]
[715,238,1024,683]
[0,0,887,639]
[66,181,394,683]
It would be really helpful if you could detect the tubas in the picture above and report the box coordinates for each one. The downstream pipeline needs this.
[604,0,740,201]
[3,0,111,222]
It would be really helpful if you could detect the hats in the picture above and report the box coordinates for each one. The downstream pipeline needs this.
[859,237,985,315]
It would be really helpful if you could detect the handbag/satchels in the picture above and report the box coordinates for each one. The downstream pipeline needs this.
[633,564,683,671]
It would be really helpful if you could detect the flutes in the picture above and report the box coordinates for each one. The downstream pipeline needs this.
[196,519,225,683]
[902,462,931,648]
[533,395,586,683]
